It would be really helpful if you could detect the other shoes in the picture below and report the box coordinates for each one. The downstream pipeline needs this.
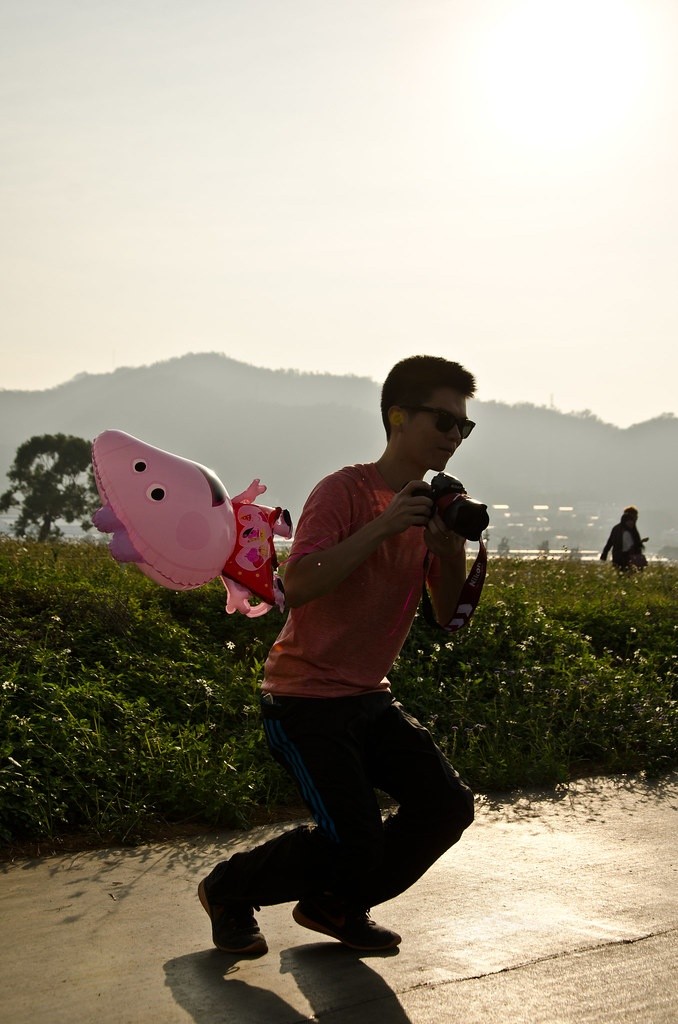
[198,880,268,954]
[292,902,401,950]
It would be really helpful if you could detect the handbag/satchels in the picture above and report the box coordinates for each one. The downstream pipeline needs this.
[630,549,647,566]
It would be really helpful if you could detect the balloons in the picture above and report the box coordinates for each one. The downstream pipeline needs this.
[90,429,297,618]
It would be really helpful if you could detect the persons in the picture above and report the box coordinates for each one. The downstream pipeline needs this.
[196,354,475,957]
[600,506,650,574]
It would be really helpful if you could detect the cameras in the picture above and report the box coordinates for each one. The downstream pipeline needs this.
[409,472,489,542]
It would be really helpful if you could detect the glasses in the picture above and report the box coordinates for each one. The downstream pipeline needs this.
[403,404,476,438]
